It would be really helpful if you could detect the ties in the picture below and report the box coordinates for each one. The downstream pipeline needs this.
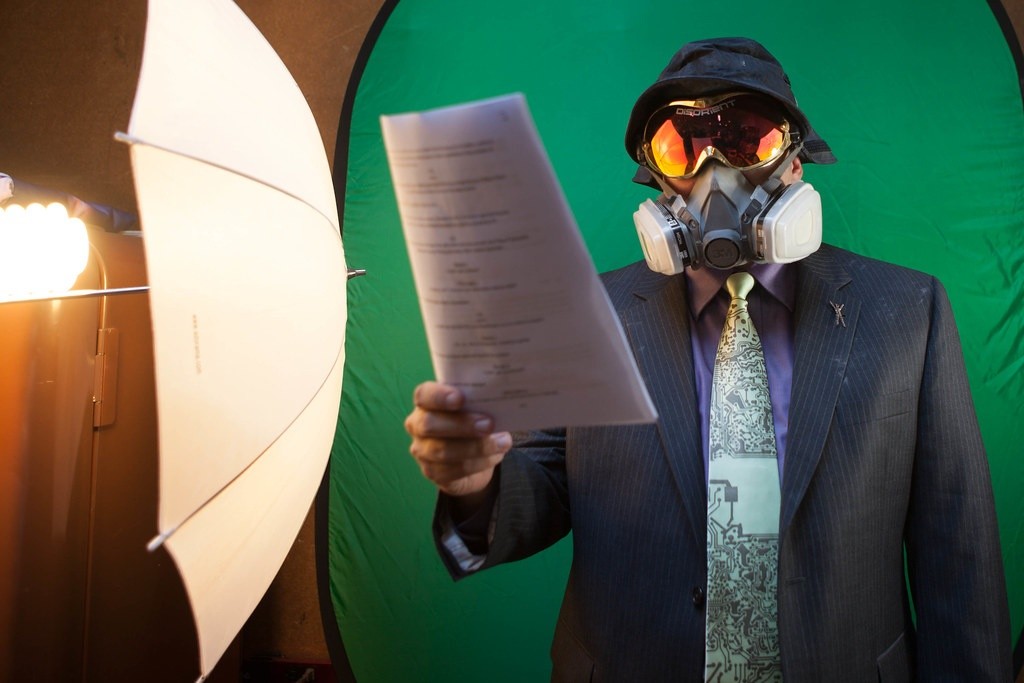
[705,270,783,683]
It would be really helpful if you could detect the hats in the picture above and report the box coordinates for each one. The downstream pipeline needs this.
[623,37,837,165]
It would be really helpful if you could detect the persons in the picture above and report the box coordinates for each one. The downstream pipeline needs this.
[401,36,1024,683]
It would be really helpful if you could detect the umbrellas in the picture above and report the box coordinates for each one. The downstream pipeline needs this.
[2,0,369,682]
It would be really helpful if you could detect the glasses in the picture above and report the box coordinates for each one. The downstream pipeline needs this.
[641,86,790,179]
